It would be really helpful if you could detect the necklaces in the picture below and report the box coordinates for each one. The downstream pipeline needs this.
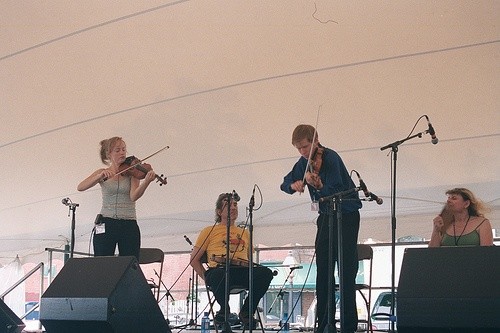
[454,215,470,246]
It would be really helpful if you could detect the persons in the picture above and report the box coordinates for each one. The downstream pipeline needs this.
[280,125,362,333]
[429,188,493,247]
[78,137,155,259]
[190,193,272,328]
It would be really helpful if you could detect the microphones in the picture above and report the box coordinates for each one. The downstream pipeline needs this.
[290,265,303,270]
[62,197,69,203]
[426,117,439,144]
[357,173,369,197]
[367,191,383,205]
[248,189,256,206]
[232,190,240,202]
[184,236,192,245]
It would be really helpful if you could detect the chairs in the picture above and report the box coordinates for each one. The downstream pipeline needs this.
[200,250,264,333]
[314,244,373,333]
[139,248,164,302]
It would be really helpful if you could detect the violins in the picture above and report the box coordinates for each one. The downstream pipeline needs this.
[213,257,260,267]
[306,146,325,193]
[122,155,167,186]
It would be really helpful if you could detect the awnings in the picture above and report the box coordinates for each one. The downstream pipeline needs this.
[260,262,363,289]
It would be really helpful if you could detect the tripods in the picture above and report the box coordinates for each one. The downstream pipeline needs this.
[171,245,202,333]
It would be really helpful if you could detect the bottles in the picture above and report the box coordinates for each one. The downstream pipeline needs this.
[201,312,210,333]
[281,313,289,333]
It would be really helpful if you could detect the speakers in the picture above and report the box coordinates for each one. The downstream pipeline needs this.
[398,245,500,333]
[0,299,25,333]
[40,255,172,333]
